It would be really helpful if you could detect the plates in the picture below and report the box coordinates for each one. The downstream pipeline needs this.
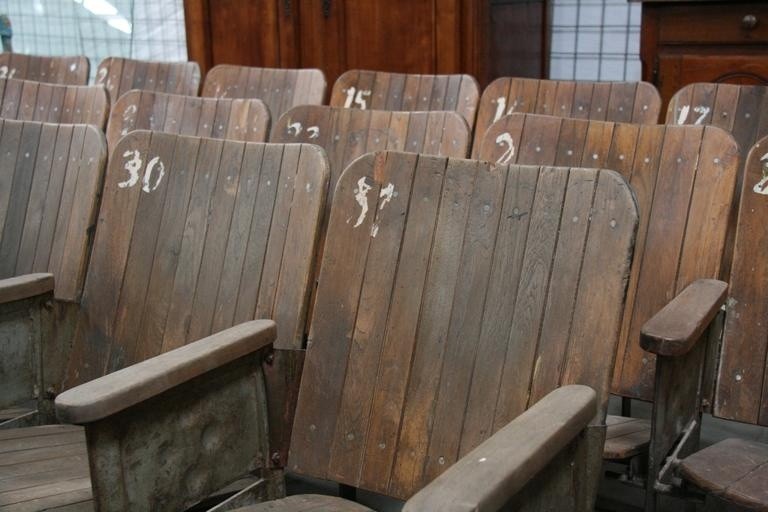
[629,0,768,126]
[186,0,552,87]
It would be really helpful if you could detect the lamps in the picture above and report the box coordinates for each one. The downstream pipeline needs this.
[663,84,768,148]
[639,134,768,508]
[329,69,474,133]
[107,91,262,161]
[0,120,102,419]
[477,77,661,134]
[57,153,639,508]
[270,106,462,339]
[202,65,325,116]
[0,77,108,132]
[95,60,199,101]
[0,130,327,510]
[0,52,88,86]
[471,116,736,508]
